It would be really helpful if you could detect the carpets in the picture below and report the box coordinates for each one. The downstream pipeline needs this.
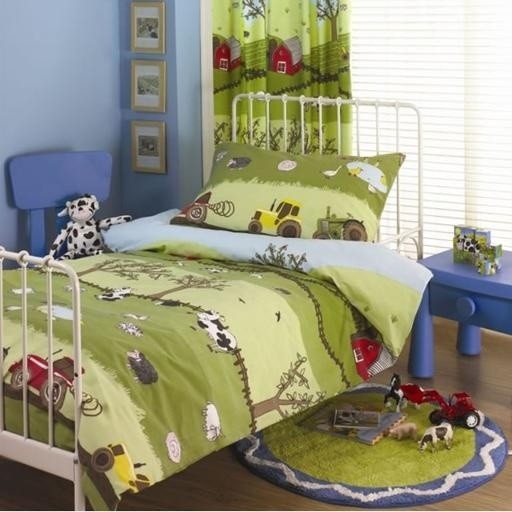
[231,382,508,509]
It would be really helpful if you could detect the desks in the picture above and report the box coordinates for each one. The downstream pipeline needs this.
[407,242,512,380]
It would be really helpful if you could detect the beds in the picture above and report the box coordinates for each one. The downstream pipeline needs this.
[0,90,434,510]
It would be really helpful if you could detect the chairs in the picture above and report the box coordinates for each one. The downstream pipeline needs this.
[9,150,115,264]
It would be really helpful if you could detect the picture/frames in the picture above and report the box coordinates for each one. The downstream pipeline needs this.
[130,120,167,175]
[130,2,165,54]
[131,59,166,113]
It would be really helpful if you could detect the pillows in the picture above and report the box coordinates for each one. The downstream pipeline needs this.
[170,141,408,244]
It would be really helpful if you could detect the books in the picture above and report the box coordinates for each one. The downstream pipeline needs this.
[331,407,381,430]
[295,401,409,446]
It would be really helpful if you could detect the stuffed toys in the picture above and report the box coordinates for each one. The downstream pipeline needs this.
[46,194,132,262]
